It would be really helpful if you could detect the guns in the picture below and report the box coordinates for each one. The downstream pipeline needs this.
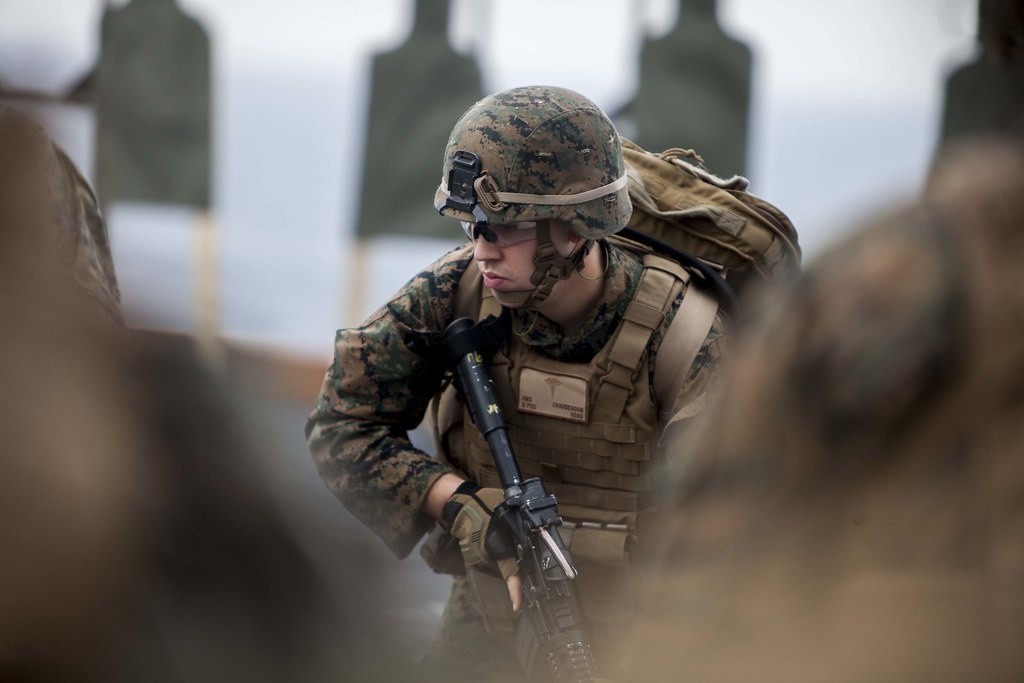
[402,315,604,681]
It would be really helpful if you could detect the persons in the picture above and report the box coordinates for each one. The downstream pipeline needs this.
[0,85,440,683]
[306,87,732,683]
[622,137,1024,683]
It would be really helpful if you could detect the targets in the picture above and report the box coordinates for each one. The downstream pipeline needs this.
[615,0,752,182]
[88,0,213,212]
[350,0,487,238]
[934,0,1024,172]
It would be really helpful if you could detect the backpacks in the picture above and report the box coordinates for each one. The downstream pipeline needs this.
[616,135,803,432]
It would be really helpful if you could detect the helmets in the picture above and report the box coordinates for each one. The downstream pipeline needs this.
[433,86,634,239]
